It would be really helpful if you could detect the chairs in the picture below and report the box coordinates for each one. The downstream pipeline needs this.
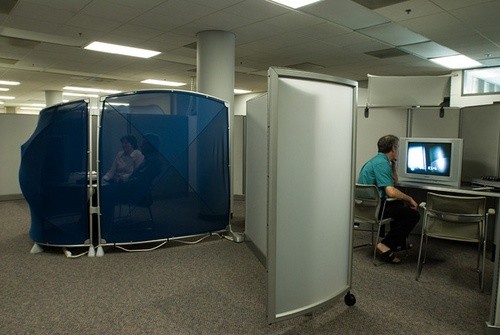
[354,183,401,266]
[111,188,155,231]
[415,192,488,290]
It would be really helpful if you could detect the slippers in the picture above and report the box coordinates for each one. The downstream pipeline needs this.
[376,243,402,264]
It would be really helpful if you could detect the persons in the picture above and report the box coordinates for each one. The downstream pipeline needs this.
[91,137,161,244]
[357,135,422,264]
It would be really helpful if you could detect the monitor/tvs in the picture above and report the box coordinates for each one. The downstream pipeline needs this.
[398,137,463,189]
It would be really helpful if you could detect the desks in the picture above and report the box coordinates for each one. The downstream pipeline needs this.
[60,182,112,187]
[395,180,500,197]
